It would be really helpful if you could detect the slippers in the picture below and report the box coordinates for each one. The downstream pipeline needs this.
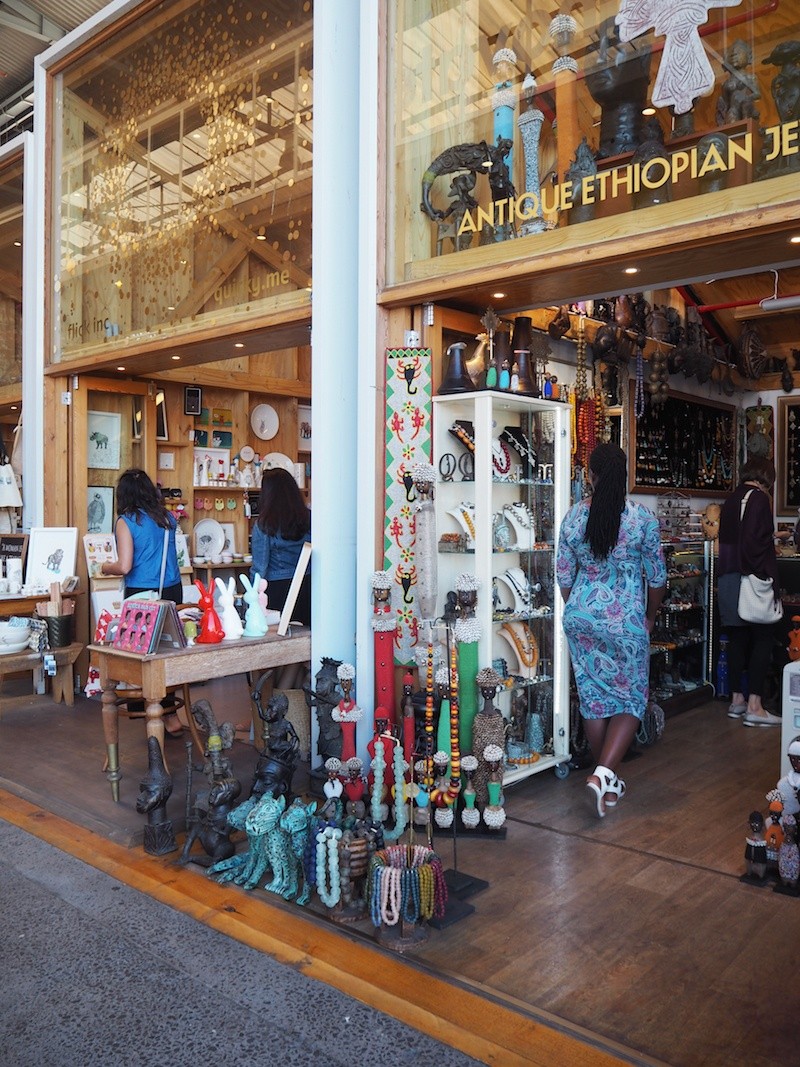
[162,716,185,739]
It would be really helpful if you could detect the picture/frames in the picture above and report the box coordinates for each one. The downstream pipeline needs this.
[89,486,115,533]
[298,404,312,452]
[193,447,230,476]
[184,386,202,417]
[87,408,122,470]
[25,527,79,587]
[219,520,236,555]
[133,387,170,441]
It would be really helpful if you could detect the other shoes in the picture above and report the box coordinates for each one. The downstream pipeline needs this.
[727,702,748,718]
[234,720,251,732]
[742,709,782,727]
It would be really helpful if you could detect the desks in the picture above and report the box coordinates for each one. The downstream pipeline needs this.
[0,591,86,617]
[86,626,312,802]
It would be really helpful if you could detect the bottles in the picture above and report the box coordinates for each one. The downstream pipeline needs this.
[614,295,632,327]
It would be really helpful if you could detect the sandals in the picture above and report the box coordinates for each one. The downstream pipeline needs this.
[584,766,614,818]
[604,774,626,806]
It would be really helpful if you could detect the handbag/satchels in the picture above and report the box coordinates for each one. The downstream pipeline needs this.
[738,574,783,624]
[120,589,160,613]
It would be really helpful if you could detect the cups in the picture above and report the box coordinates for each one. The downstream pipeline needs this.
[33,608,75,647]
[0,577,21,594]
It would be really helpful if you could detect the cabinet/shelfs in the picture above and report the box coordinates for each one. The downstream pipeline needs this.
[440,389,573,796]
[578,538,800,752]
[74,366,312,579]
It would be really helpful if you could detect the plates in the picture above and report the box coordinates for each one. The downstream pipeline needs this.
[194,518,225,556]
[261,452,295,478]
[251,403,279,441]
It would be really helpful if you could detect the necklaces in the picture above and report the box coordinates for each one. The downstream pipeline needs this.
[451,423,540,764]
[424,625,462,808]
[304,734,449,926]
[529,318,736,536]
[534,545,664,769]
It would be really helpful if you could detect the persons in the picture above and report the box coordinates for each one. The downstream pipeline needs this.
[102,468,183,738]
[442,16,800,251]
[717,455,790,727]
[745,736,800,889]
[250,468,311,626]
[135,657,393,867]
[557,443,667,818]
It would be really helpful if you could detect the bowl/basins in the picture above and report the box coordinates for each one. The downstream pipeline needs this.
[0,621,31,655]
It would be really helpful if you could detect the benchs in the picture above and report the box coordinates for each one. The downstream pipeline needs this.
[0,642,84,707]
